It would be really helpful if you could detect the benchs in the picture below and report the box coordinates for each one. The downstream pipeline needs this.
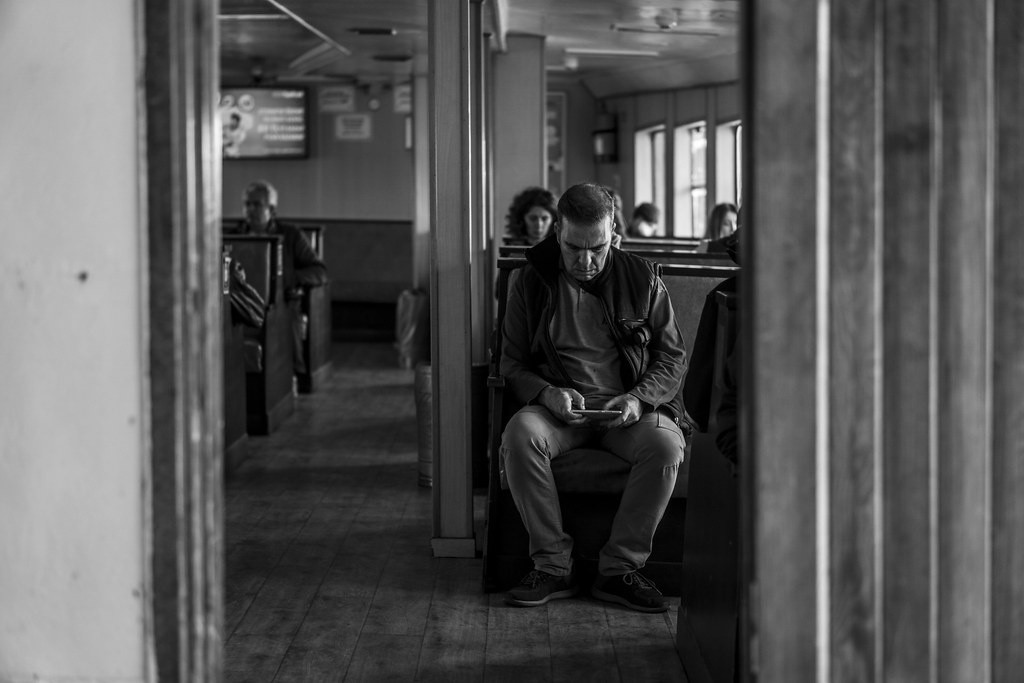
[218,218,329,467]
[496,232,738,583]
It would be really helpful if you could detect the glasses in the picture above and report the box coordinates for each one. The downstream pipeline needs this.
[243,200,268,208]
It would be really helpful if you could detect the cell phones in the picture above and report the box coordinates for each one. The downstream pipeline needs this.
[571,409,622,420]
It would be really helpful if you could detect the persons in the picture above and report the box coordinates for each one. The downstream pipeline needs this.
[234,180,332,375]
[625,203,662,236]
[505,186,561,250]
[709,203,740,242]
[496,184,691,615]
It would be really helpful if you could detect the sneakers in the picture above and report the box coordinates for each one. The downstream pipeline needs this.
[592,568,669,614]
[505,569,575,608]
